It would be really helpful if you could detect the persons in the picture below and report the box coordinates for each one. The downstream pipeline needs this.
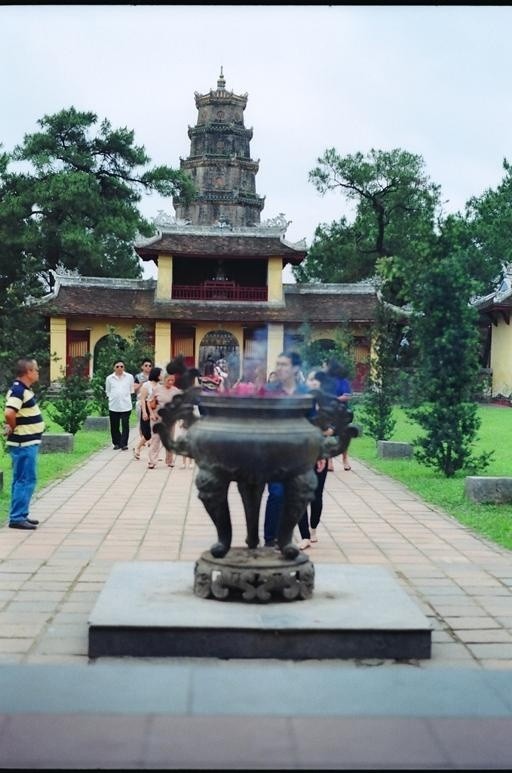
[100,343,358,477]
[258,348,322,552]
[3,356,47,530]
[295,367,330,552]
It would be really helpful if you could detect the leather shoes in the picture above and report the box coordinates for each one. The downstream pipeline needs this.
[8,517,39,529]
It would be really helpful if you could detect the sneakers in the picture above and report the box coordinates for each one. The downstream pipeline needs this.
[113,444,192,470]
[298,528,319,550]
[327,463,352,471]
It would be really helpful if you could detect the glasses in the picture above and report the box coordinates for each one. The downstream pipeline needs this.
[145,365,151,367]
[115,366,123,369]
